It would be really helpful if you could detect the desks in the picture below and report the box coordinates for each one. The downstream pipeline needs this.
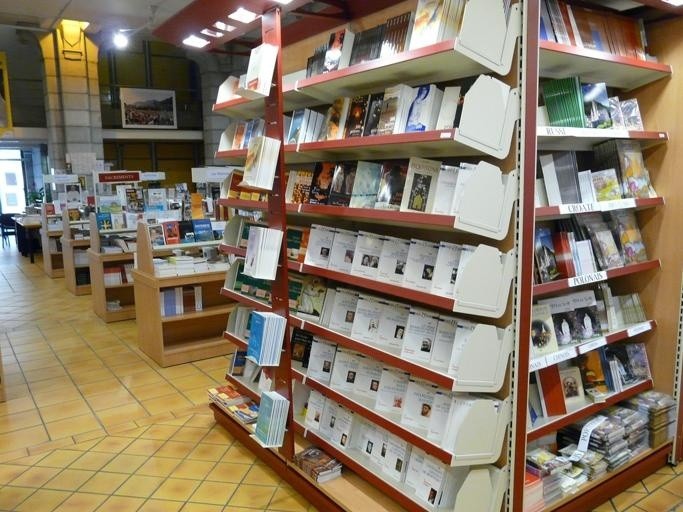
[10,213,42,264]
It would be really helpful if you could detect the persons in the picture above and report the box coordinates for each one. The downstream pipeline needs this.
[124,106,174,125]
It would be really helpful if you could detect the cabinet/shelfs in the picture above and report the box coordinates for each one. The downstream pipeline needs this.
[42,201,238,370]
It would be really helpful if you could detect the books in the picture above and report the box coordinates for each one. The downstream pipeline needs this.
[216,1,680,510]
[23,152,214,318]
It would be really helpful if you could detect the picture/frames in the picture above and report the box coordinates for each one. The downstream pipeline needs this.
[119,85,178,130]
